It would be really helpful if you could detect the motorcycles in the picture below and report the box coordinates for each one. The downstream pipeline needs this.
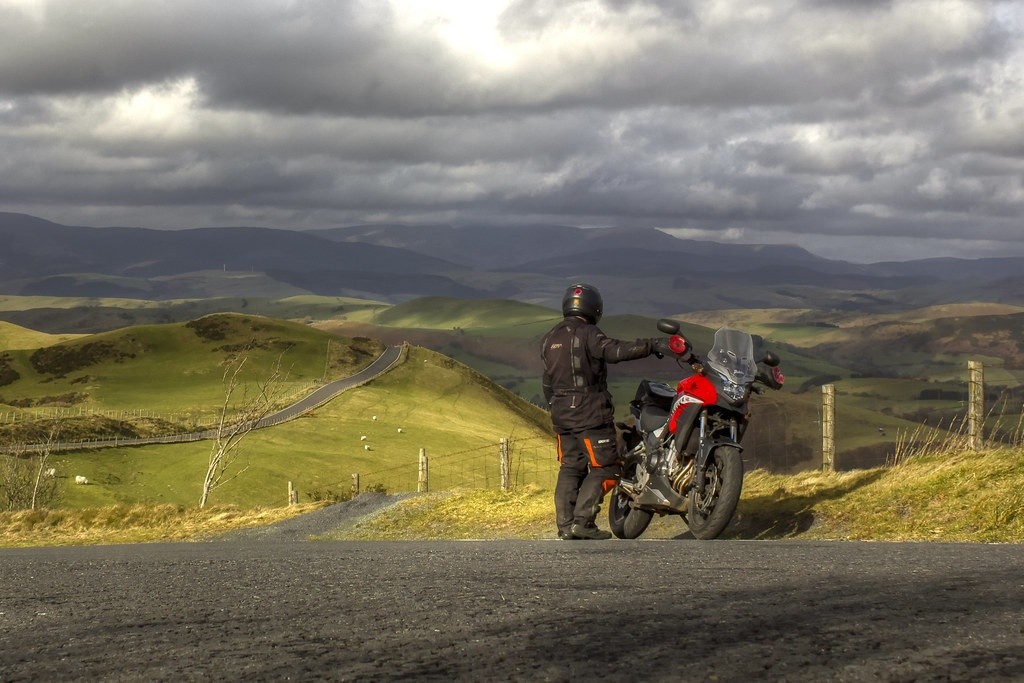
[608,317,786,540]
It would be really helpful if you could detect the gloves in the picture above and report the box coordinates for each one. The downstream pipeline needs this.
[653,337,692,362]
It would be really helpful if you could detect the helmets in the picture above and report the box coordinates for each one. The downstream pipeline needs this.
[562,283,603,325]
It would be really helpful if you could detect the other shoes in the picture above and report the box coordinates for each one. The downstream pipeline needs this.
[571,524,612,539]
[557,530,574,540]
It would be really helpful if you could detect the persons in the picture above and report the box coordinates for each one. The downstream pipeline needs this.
[540,282,692,541]
